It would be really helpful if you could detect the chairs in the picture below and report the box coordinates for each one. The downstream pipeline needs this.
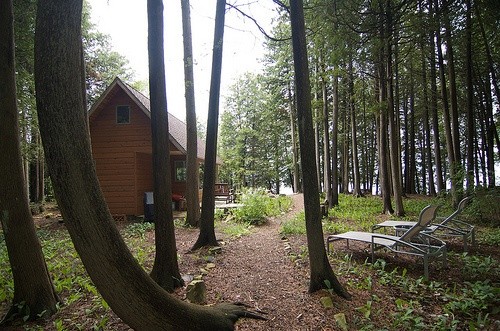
[371,196,477,251]
[325,205,448,282]
